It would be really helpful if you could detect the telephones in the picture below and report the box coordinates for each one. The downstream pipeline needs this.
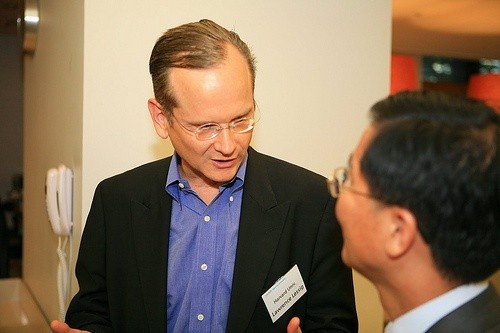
[44,162,74,236]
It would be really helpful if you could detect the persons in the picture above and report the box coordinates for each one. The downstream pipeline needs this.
[327,90,500,333]
[51,19,358,333]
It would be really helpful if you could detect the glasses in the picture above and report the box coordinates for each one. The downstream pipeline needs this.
[327,166,407,208]
[160,100,261,140]
